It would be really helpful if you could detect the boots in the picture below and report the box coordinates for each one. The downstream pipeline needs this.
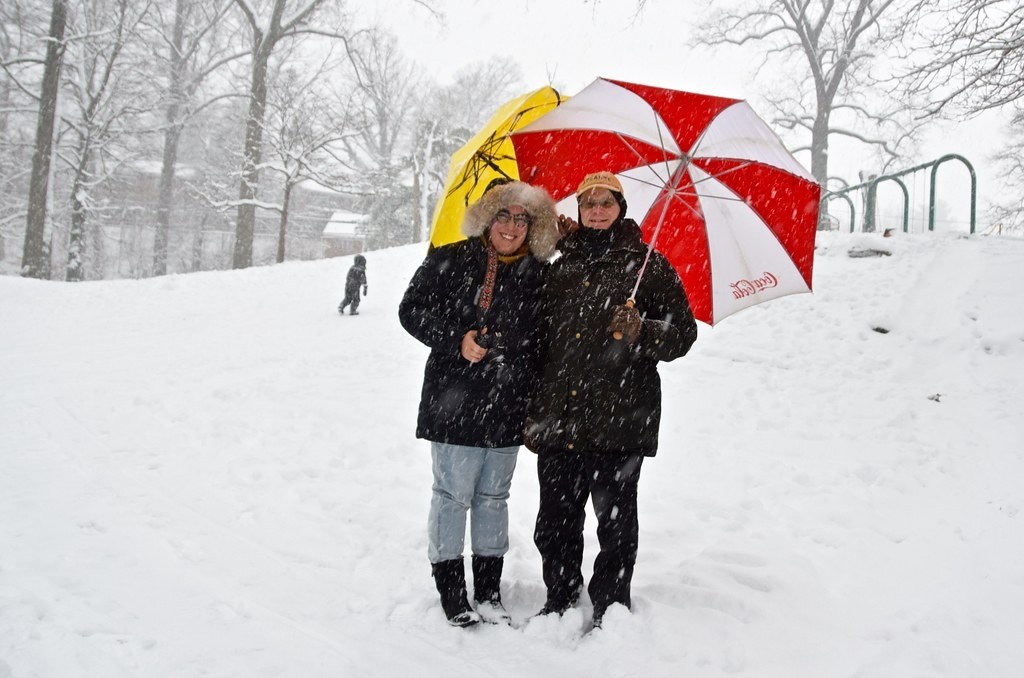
[430,556,480,629]
[470,554,513,626]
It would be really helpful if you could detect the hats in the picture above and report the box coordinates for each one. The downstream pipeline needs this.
[575,171,625,199]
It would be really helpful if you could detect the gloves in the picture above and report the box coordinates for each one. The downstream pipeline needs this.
[363,285,368,296]
[522,415,550,453]
[605,305,643,343]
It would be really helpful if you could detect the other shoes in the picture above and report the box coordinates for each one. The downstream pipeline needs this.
[350,312,359,315]
[338,306,344,314]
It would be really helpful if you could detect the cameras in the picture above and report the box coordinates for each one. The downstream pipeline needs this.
[475,331,503,350]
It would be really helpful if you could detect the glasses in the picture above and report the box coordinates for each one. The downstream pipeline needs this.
[496,210,531,228]
[580,197,618,209]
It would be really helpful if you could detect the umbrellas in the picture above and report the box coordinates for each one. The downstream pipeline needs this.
[427,86,578,248]
[510,76,823,339]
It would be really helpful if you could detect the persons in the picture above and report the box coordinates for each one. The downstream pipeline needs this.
[522,170,697,631]
[397,177,560,630]
[338,254,368,316]
